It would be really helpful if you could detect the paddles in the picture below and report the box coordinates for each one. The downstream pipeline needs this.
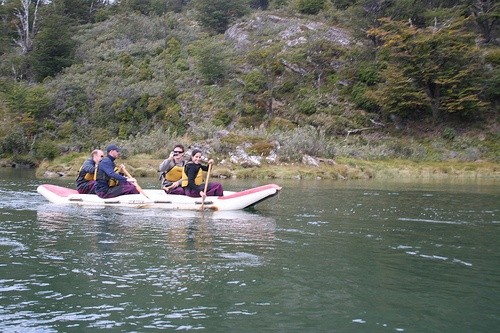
[121,167,152,201]
[200,164,212,212]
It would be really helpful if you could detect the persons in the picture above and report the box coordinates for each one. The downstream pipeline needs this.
[94,145,140,199]
[76,149,104,194]
[159,144,188,195]
[182,149,223,198]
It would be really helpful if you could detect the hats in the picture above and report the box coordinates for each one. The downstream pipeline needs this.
[106,145,122,152]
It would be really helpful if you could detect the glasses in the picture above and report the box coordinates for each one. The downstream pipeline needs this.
[173,150,183,153]
[101,156,104,157]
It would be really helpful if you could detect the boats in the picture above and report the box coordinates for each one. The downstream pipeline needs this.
[36,181,283,212]
